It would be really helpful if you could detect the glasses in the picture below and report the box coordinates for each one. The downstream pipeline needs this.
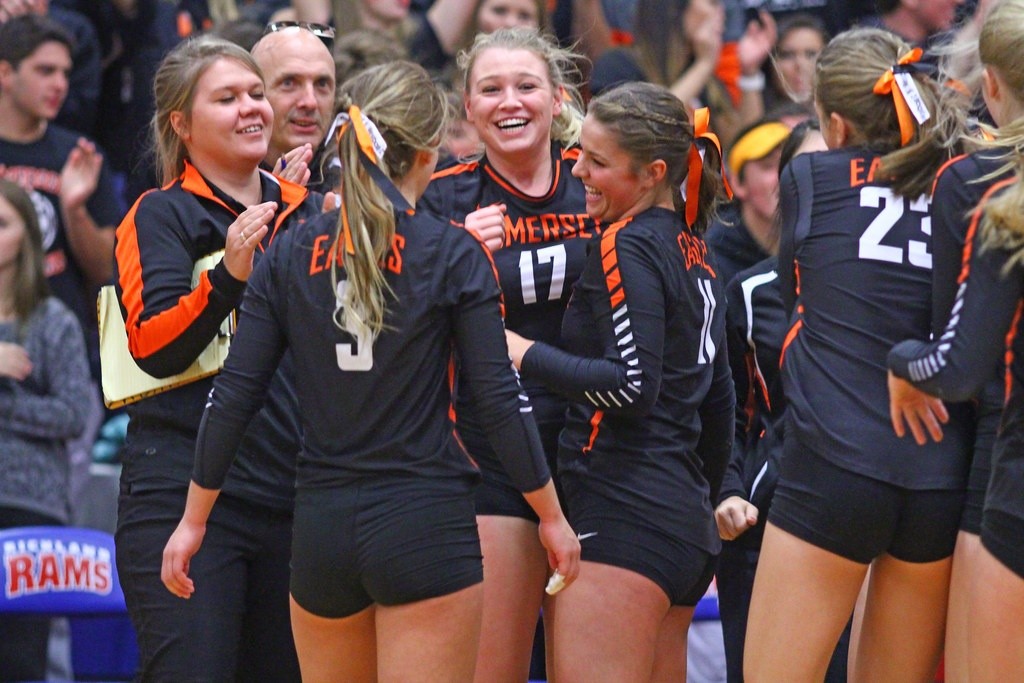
[263,21,337,50]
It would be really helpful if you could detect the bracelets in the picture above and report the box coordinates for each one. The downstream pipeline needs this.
[737,72,766,92]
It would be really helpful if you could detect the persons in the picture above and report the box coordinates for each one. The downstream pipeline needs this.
[1,176,92,683]
[113,0,779,683]
[709,0,1024,683]
[0,2,128,386]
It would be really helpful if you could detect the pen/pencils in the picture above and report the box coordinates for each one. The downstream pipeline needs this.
[281,151,287,169]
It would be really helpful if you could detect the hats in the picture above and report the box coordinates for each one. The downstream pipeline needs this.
[728,122,793,178]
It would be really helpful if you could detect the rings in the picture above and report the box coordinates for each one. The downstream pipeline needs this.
[239,231,247,241]
